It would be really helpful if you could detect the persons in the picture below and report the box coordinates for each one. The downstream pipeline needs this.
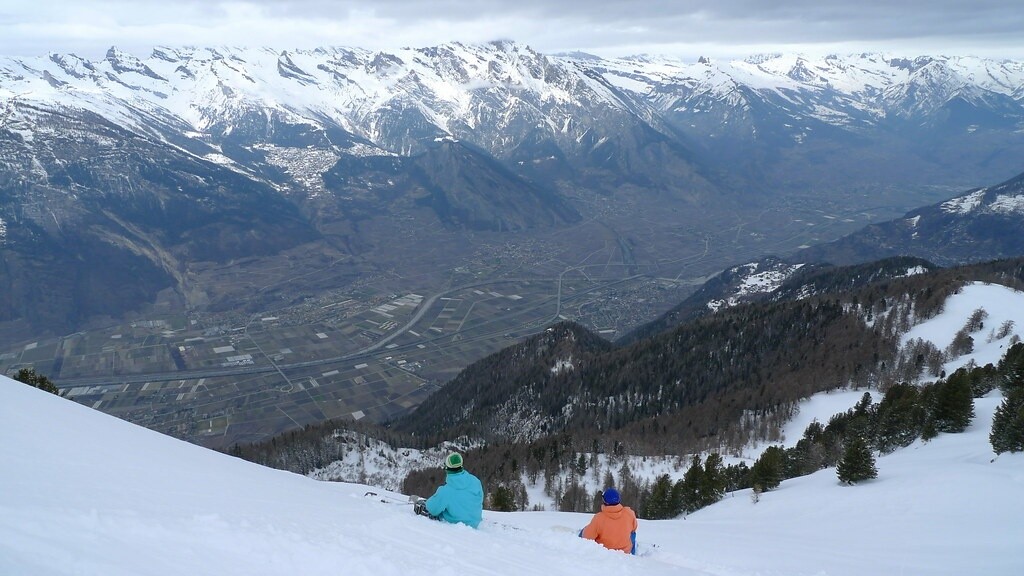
[579,489,638,555]
[414,452,484,529]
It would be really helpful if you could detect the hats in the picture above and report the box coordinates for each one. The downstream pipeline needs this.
[446,452,463,469]
[603,488,620,505]
